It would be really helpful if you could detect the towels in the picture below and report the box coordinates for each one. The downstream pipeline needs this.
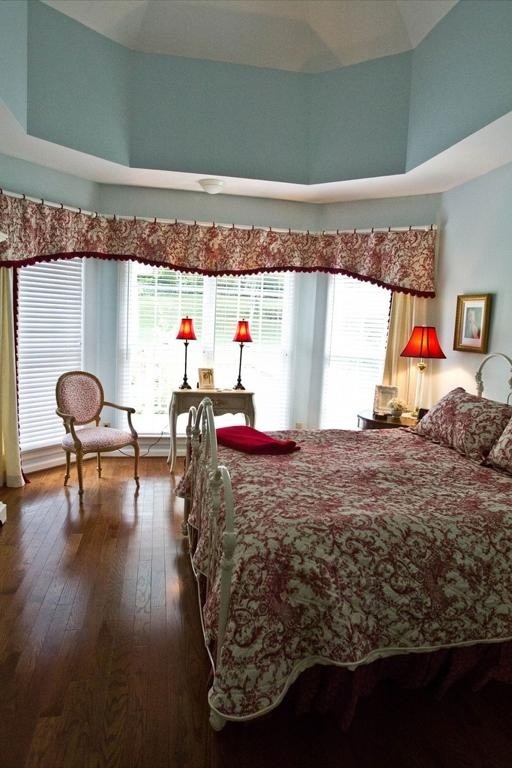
[214,425,300,455]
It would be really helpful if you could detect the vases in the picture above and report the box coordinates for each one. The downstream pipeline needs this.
[390,409,402,420]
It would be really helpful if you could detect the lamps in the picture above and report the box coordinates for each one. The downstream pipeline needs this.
[400,325,448,420]
[198,178,227,195]
[232,318,252,390]
[175,315,197,389]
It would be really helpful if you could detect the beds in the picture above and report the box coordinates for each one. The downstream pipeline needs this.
[178,353,512,740]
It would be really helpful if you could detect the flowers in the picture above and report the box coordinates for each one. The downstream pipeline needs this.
[386,397,410,411]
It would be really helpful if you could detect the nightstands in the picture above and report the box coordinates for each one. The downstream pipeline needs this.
[354,411,418,429]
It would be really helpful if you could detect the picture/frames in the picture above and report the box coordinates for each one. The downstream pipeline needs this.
[454,293,491,354]
[371,383,400,415]
[198,367,215,389]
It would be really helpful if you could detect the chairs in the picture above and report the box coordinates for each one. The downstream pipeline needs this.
[55,371,142,504]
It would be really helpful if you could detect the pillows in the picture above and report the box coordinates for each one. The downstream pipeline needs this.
[400,386,512,466]
[481,412,512,478]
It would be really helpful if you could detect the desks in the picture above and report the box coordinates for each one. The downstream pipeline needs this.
[165,390,255,473]
